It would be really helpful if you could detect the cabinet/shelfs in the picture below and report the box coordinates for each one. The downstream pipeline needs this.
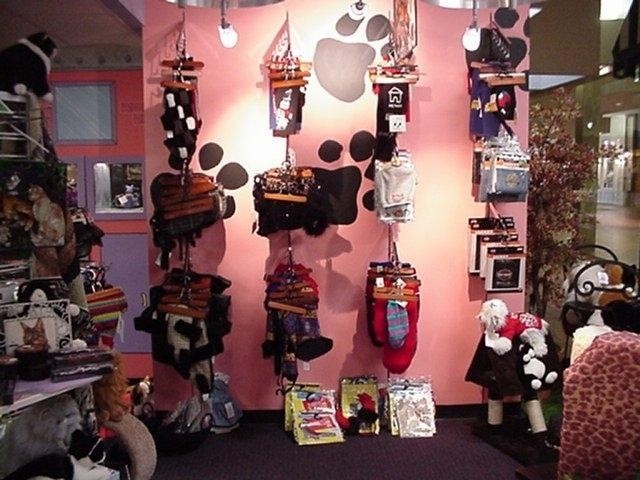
[90,220,154,378]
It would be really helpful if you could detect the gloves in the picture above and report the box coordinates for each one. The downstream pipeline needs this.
[367,260,421,375]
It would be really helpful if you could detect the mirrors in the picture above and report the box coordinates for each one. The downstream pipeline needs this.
[54,155,87,208]
[85,155,147,220]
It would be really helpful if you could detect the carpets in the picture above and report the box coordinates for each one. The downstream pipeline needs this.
[472,410,562,467]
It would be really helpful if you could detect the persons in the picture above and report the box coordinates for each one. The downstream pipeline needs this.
[494,261,516,288]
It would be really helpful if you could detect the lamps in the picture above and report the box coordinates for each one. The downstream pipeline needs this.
[217,0,238,48]
[347,0,365,23]
[461,1,482,51]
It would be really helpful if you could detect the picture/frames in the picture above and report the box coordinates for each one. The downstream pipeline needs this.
[0,298,72,366]
[50,79,118,145]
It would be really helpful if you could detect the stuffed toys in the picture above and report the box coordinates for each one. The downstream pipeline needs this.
[515,341,558,391]
[0,383,135,480]
[475,298,552,357]
[0,30,59,103]
[561,258,640,336]
